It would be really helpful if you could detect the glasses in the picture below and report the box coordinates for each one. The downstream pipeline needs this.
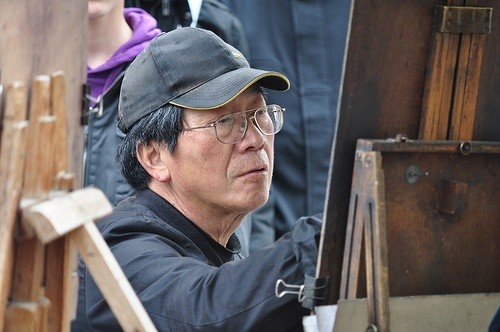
[174,103,286,144]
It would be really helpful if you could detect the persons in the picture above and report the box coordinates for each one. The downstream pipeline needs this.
[84,0,194,209]
[70,27,325,332]
[194,0,350,253]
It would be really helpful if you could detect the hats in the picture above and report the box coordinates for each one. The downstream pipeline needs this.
[116,27,291,133]
